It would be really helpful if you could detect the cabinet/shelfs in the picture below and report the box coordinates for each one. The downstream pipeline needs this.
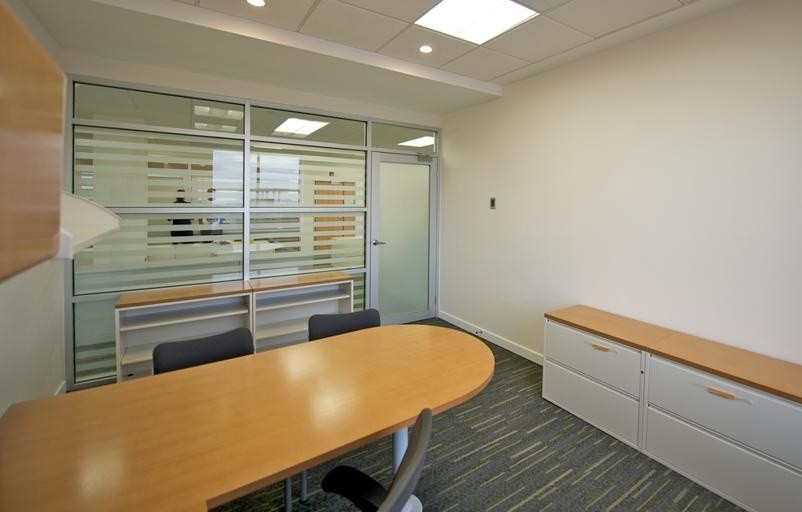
[247,271,354,353]
[115,281,253,382]
[641,327,801,512]
[541,305,677,450]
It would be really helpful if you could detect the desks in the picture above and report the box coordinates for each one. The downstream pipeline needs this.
[1,324,496,511]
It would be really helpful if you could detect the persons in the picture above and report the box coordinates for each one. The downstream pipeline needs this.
[198,188,226,244]
[167,189,195,244]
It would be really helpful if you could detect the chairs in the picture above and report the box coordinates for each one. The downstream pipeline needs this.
[300,309,381,500]
[153,327,293,510]
